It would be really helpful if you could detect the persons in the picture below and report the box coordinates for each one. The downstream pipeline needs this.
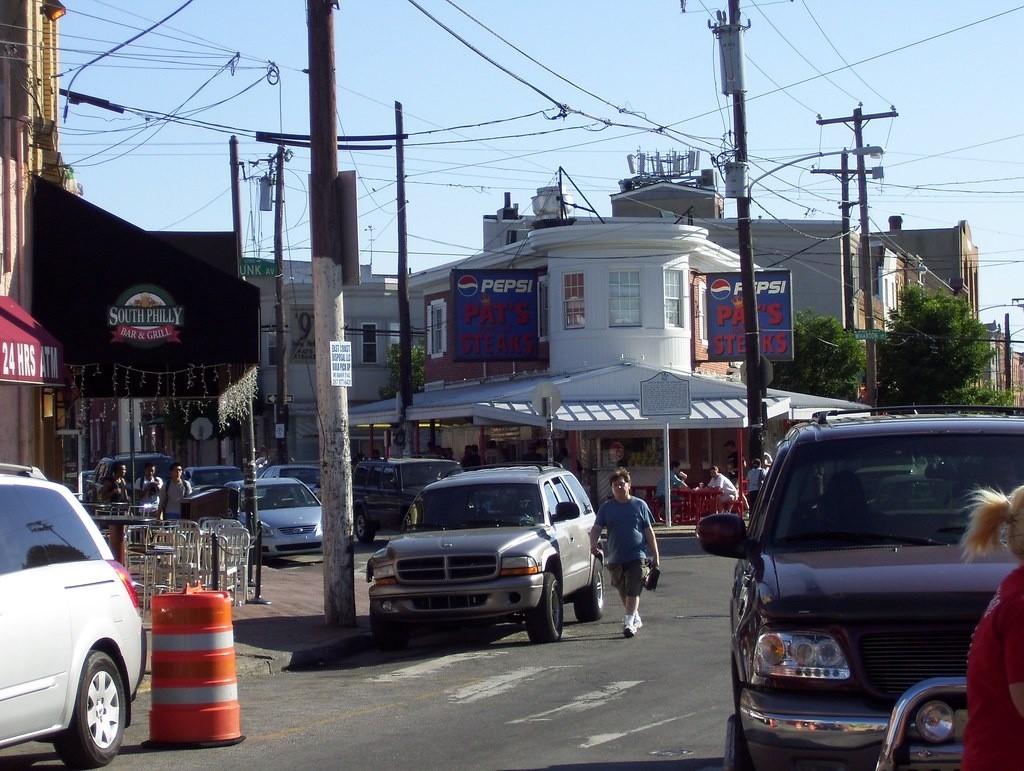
[383,472,395,482]
[258,486,295,510]
[590,467,659,638]
[951,480,1024,771]
[723,440,746,489]
[102,460,129,503]
[427,439,584,479]
[708,466,738,513]
[354,468,367,485]
[746,459,768,520]
[134,463,164,516]
[762,449,773,473]
[655,461,689,522]
[154,462,193,530]
[351,449,381,476]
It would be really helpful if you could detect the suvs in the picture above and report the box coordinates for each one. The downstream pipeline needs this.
[351,454,502,544]
[364,461,605,659]
[84,451,178,504]
[696,402,1024,770]
[0,464,147,771]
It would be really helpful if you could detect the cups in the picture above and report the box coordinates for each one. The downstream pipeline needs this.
[699,483,704,490]
[679,471,687,479]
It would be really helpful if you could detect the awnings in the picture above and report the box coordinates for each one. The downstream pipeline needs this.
[31,173,262,398]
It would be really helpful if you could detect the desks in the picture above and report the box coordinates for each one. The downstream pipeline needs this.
[91,515,157,567]
[680,488,705,524]
[630,485,655,498]
[81,503,112,515]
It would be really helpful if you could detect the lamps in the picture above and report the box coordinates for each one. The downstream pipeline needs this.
[38,0,69,22]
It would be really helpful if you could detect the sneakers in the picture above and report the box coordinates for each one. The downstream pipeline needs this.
[623,625,636,638]
[634,615,643,630]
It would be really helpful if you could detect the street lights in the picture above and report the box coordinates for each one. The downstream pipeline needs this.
[844,265,928,403]
[736,145,885,470]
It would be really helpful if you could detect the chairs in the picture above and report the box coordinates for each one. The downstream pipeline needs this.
[607,480,750,527]
[94,504,254,621]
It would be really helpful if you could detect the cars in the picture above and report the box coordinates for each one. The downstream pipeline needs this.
[853,450,928,486]
[223,478,324,565]
[183,465,244,487]
[258,464,323,495]
